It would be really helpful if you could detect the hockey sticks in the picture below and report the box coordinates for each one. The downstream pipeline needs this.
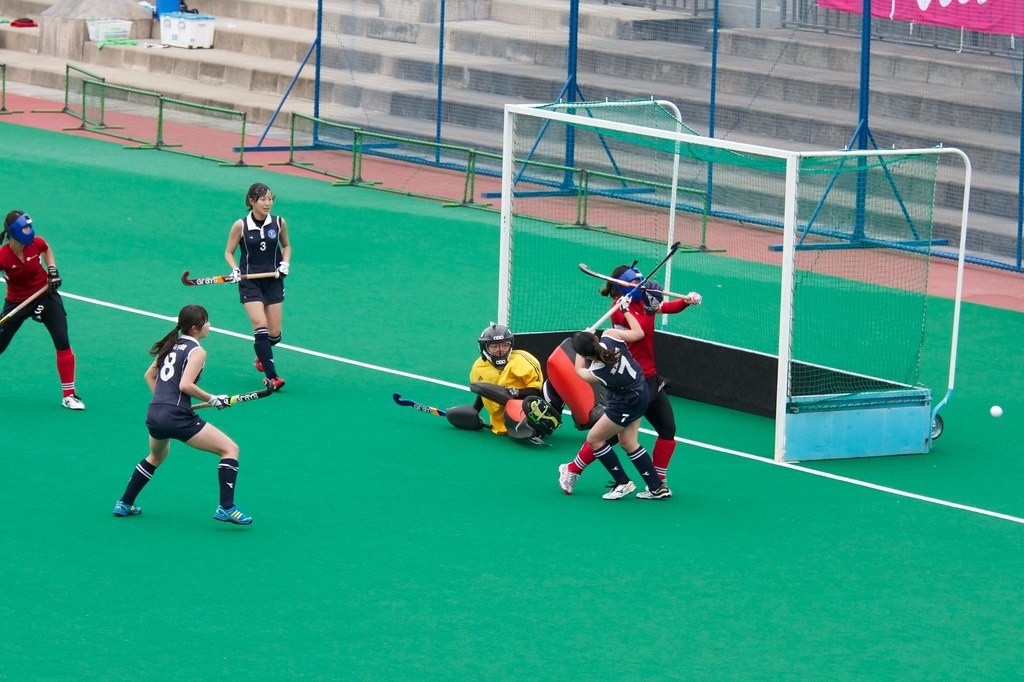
[190,378,274,410]
[589,240,681,331]
[0,284,48,325]
[181,270,276,286]
[578,263,691,301]
[393,392,544,446]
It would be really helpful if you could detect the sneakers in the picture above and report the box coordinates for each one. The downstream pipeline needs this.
[636,483,672,499]
[645,480,668,491]
[269,377,285,391]
[254,357,264,373]
[61,394,84,410]
[213,505,252,525]
[112,500,141,515]
[602,481,636,499]
[559,462,581,494]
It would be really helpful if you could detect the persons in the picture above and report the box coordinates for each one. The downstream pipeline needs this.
[572,296,671,499]
[559,261,702,495]
[113,305,253,525]
[0,211,84,409]
[225,183,291,390]
[447,325,565,447]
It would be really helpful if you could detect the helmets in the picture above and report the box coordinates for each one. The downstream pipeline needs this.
[477,321,514,370]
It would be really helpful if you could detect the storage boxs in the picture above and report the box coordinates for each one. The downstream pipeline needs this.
[160,12,215,49]
[85,20,133,41]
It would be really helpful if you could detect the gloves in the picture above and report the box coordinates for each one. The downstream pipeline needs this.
[275,262,290,280]
[47,268,62,292]
[208,395,232,410]
[229,268,241,284]
[684,292,702,306]
[581,327,596,335]
[615,296,633,314]
[641,281,664,315]
